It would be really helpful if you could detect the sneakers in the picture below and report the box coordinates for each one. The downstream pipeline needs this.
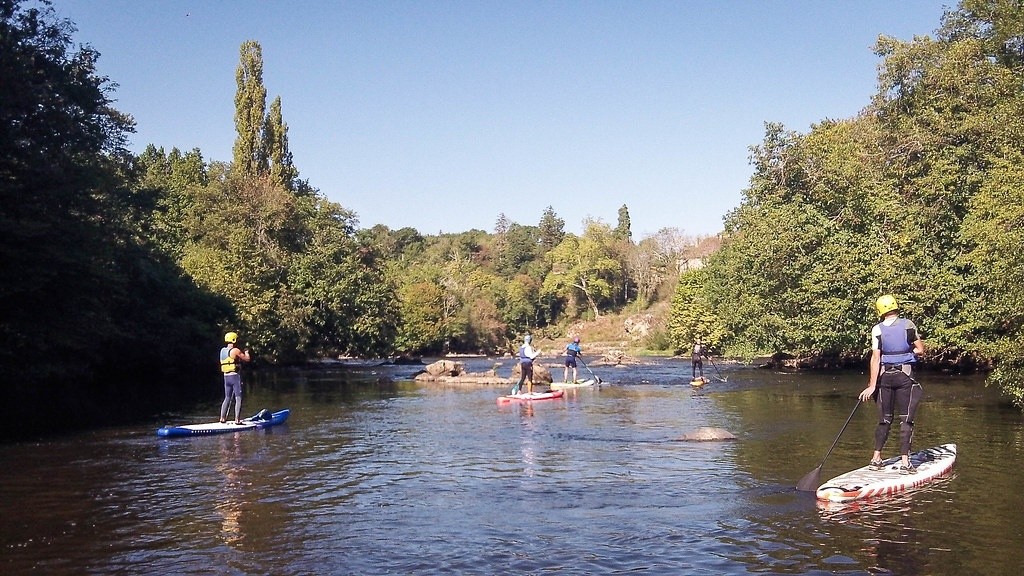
[868,459,885,470]
[898,461,917,475]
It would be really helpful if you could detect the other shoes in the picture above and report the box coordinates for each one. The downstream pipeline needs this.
[220,419,226,423]
[529,392,535,396]
[234,422,246,425]
[701,377,703,381]
[573,381,579,384]
[517,390,524,394]
[692,378,695,382]
[564,379,567,383]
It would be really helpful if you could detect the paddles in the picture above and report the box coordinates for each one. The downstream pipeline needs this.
[711,359,726,380]
[225,390,234,420]
[577,355,603,385]
[794,395,863,492]
[510,357,536,396]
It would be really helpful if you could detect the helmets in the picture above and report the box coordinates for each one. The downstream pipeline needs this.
[224,332,239,344]
[524,336,532,343]
[875,295,898,318]
[574,337,580,342]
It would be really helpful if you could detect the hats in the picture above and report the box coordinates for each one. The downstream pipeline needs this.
[695,339,701,344]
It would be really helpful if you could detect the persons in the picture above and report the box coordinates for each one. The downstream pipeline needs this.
[858,294,924,475]
[518,335,541,395]
[689,338,712,381]
[220,332,250,425]
[342,347,351,357]
[559,338,583,383]
